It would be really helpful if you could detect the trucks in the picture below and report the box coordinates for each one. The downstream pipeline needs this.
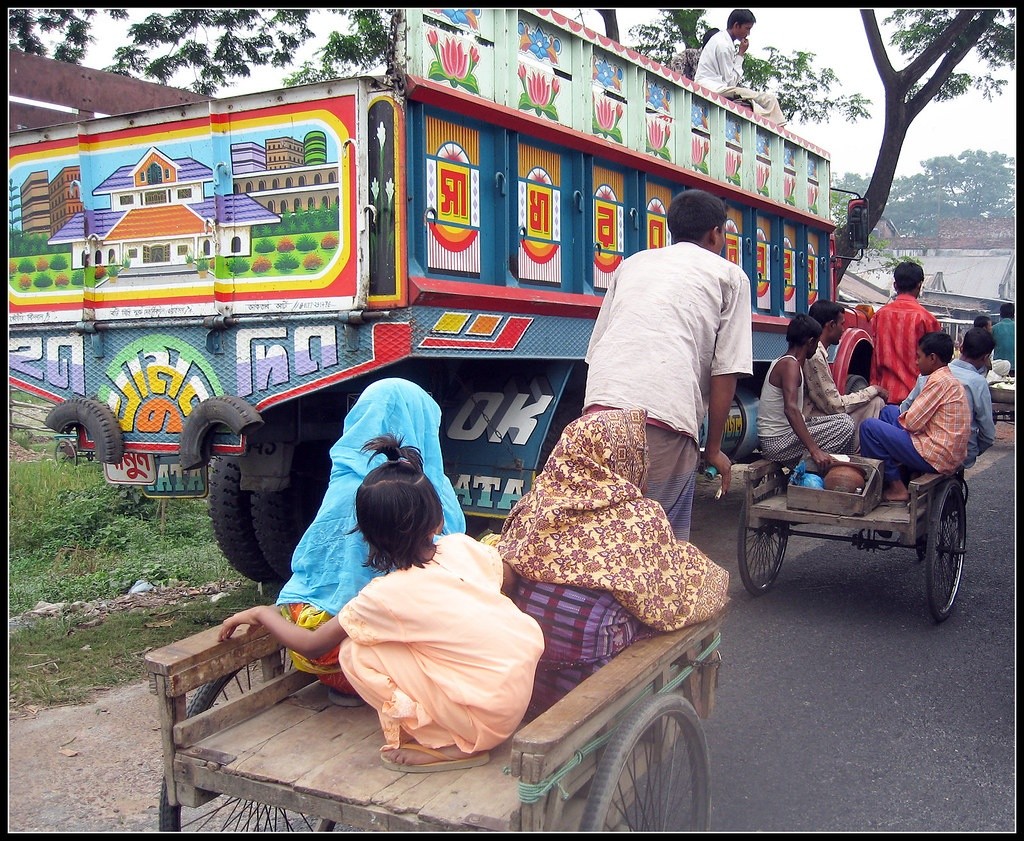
[8,7,870,576]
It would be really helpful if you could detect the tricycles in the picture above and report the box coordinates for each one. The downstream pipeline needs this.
[144,452,742,833]
[737,457,968,623]
[985,372,1016,427]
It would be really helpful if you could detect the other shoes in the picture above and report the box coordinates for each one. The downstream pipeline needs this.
[322,684,365,707]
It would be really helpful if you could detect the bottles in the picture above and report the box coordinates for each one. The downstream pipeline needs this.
[823,462,867,494]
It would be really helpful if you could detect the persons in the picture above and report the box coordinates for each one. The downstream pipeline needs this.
[217,433,545,773]
[873,262,941,406]
[666,28,720,81]
[582,189,753,542]
[990,303,1015,374]
[974,316,1011,382]
[859,327,996,507]
[694,9,787,130]
[495,409,729,723]
[274,377,466,695]
[757,300,889,479]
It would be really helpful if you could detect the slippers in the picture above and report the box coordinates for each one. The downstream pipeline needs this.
[878,493,906,507]
[379,741,490,772]
